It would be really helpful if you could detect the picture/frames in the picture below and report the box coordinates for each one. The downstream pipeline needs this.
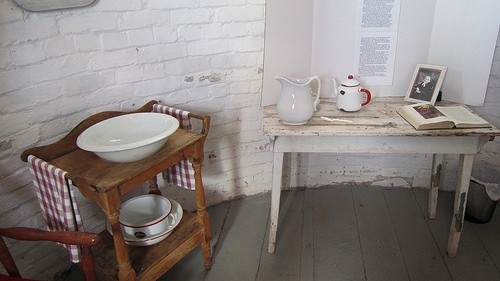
[404,63,448,106]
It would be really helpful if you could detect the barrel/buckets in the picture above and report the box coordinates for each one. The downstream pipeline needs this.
[464,163,500,223]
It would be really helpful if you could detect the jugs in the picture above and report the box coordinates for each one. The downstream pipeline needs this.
[273,75,321,124]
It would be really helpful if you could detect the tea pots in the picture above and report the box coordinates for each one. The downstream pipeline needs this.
[331,75,371,112]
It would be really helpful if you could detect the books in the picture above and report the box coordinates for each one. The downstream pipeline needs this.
[394,102,492,131]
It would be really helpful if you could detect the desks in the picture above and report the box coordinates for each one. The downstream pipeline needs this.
[263,104,500,258]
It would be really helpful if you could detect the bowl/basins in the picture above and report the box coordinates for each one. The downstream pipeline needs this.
[117,194,172,238]
[77,113,180,163]
[106,194,184,246]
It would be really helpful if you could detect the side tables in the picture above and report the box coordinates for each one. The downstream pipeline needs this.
[20,100,211,281]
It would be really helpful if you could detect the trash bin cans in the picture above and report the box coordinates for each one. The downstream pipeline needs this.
[464,165,500,223]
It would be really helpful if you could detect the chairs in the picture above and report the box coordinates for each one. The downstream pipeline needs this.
[0,227,100,281]
